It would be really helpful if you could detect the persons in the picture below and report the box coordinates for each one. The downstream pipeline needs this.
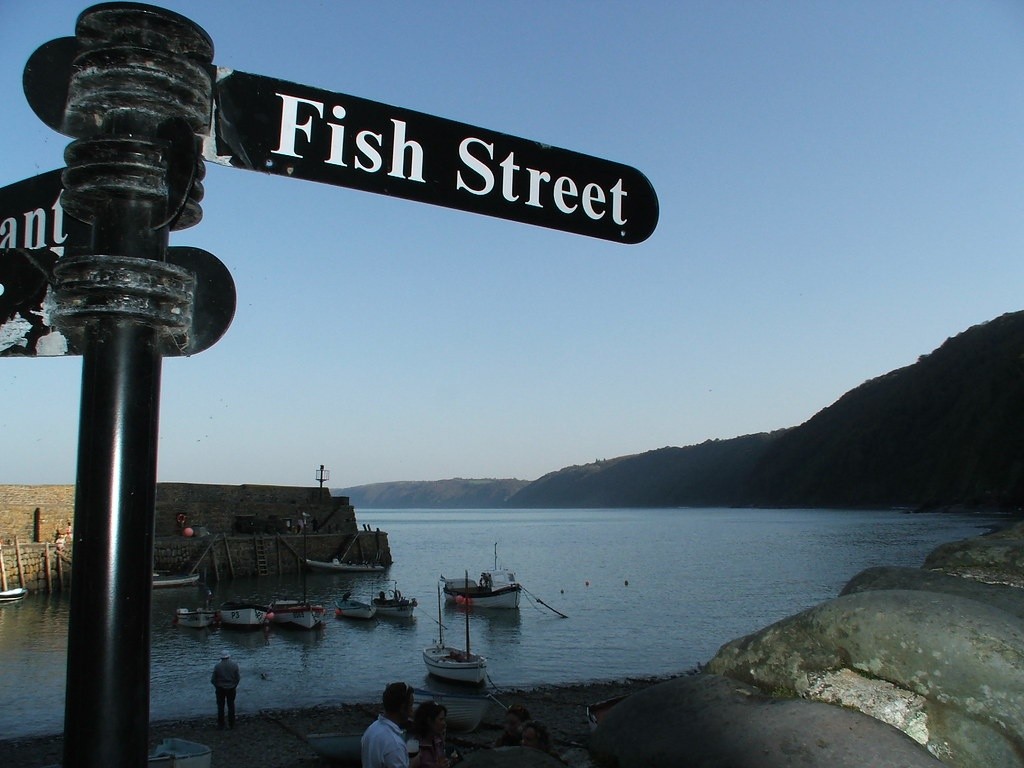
[360,683,452,768]
[310,516,319,533]
[210,650,240,729]
[297,518,304,535]
[492,705,561,763]
[55,522,72,553]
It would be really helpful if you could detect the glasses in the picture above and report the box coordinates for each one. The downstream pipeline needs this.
[403,681,410,696]
[431,702,439,708]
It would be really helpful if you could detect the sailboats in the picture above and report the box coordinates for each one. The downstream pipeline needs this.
[423,569,487,684]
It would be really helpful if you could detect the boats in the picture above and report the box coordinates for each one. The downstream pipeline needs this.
[0,588,27,605]
[333,592,378,620]
[412,688,491,734]
[148,737,213,767]
[275,576,326,631]
[305,531,386,572]
[172,600,217,628]
[440,542,525,607]
[371,579,417,617]
[306,730,419,766]
[215,594,275,630]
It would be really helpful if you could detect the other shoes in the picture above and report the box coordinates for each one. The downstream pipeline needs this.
[214,726,224,730]
[228,726,236,729]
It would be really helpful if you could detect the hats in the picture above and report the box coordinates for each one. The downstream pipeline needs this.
[219,650,231,659]
